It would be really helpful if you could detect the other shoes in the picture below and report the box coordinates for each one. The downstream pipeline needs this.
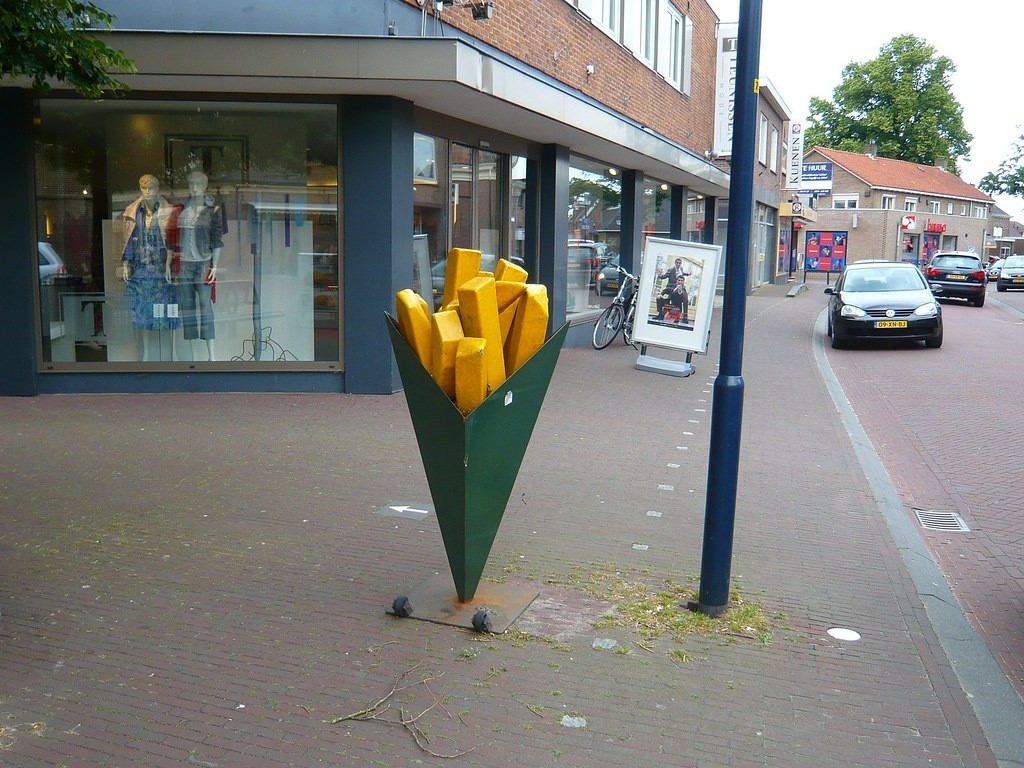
[655,317,664,320]
[683,319,688,324]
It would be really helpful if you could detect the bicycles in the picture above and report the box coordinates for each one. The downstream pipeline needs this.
[590,262,646,352]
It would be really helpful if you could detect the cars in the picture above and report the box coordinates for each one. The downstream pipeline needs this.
[996,255,1024,293]
[920,249,987,308]
[824,261,944,352]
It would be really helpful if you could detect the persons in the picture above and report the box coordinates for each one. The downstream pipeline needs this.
[807,232,846,272]
[118,171,178,362]
[652,275,686,324]
[657,258,692,324]
[172,169,226,365]
